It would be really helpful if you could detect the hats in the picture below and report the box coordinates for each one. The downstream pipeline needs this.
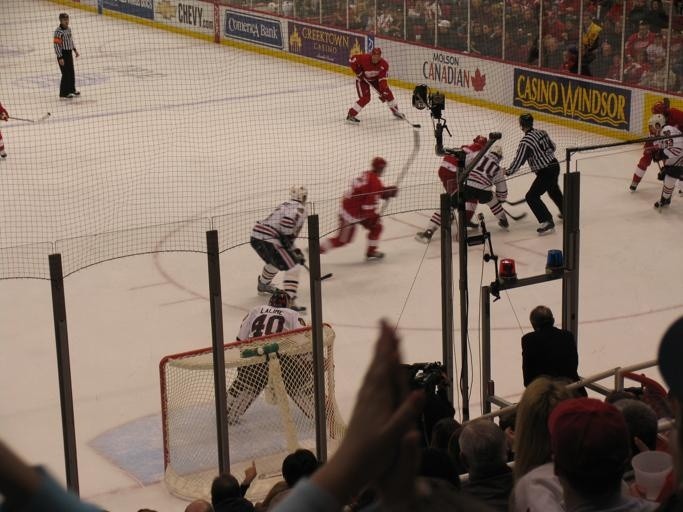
[548,397,627,464]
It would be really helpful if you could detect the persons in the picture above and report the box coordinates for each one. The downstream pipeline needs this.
[250,186,308,311]
[347,47,405,122]
[629,101,683,190]
[223,1,683,96]
[438,135,488,227]
[416,145,509,239]
[319,158,398,258]
[1,291,682,512]
[53,13,80,98]
[648,114,683,207]
[502,113,563,232]
[0,103,9,157]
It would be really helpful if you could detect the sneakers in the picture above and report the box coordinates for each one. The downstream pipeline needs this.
[367,251,384,257]
[393,111,405,119]
[63,94,73,98]
[73,91,80,95]
[467,221,478,228]
[416,229,433,238]
[654,198,671,207]
[630,185,636,190]
[498,215,509,228]
[346,112,360,122]
[536,217,555,233]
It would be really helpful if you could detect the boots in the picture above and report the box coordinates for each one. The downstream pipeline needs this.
[290,295,306,311]
[257,275,275,293]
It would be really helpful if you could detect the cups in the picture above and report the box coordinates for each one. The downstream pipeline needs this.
[631,451,674,500]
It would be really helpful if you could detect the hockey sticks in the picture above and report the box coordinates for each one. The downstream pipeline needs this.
[504,210,529,221]
[291,249,333,281]
[376,129,420,220]
[9,113,52,124]
[504,199,524,206]
[364,78,421,128]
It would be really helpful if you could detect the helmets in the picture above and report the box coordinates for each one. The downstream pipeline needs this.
[652,102,664,114]
[372,47,381,57]
[472,135,487,146]
[290,184,308,202]
[269,288,291,309]
[489,145,503,161]
[372,156,387,170]
[519,112,533,130]
[648,114,667,130]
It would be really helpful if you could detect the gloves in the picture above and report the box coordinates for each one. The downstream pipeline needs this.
[378,90,389,103]
[356,71,364,81]
[293,248,305,265]
